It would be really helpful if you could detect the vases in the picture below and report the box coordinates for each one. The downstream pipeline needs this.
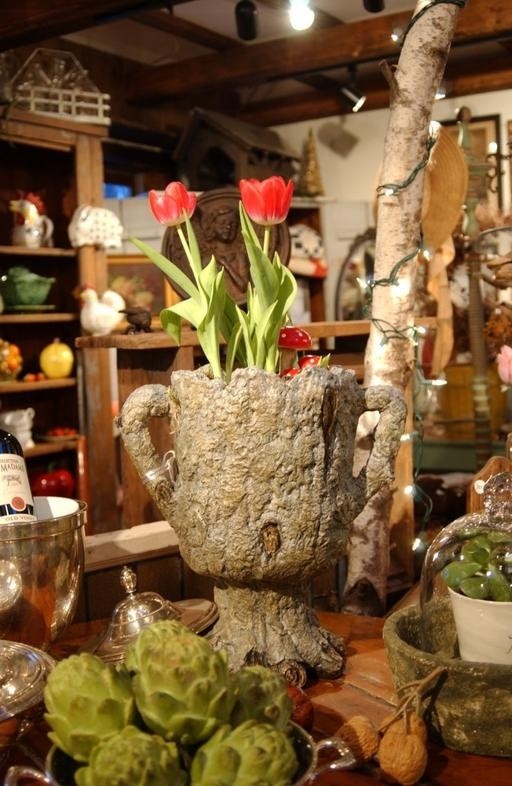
[115,364,407,694]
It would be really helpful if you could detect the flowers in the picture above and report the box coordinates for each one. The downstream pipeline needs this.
[127,177,334,377]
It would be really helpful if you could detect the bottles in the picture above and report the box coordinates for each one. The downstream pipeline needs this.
[0,421,36,529]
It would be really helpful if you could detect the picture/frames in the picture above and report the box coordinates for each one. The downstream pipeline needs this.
[439,112,502,221]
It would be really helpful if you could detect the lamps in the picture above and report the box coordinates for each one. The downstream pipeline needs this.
[233,1,446,112]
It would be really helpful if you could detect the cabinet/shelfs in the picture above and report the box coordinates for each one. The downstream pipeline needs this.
[0,106,117,535]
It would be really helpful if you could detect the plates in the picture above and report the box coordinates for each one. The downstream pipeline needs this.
[41,435,77,442]
[3,304,56,313]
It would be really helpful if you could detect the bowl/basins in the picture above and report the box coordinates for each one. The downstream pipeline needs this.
[0,268,54,302]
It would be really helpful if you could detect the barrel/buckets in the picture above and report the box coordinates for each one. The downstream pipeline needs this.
[2,499,88,654]
